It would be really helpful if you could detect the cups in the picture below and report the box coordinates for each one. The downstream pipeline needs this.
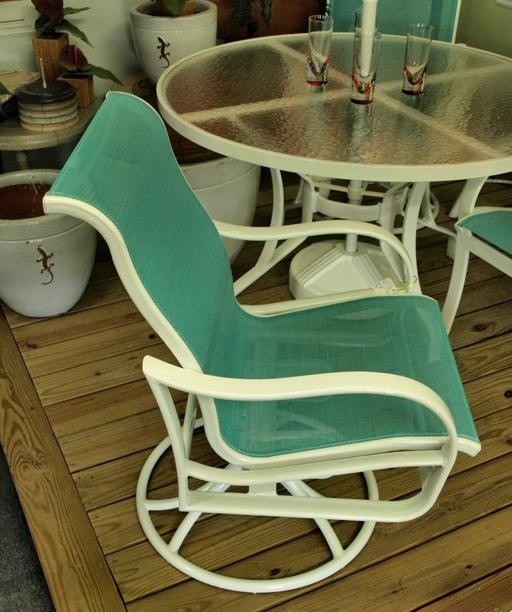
[351,27,381,102]
[401,22,433,95]
[306,14,334,85]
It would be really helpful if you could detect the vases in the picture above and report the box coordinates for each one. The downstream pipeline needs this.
[0,170,98,317]
[179,158,262,268]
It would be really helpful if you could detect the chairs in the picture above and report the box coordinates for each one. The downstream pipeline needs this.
[297,1,461,230]
[41,88,484,598]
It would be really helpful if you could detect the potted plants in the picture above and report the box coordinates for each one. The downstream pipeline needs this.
[57,43,125,108]
[29,2,96,79]
[129,1,219,85]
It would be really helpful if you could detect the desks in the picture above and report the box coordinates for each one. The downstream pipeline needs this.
[154,31,512,299]
[0,69,104,171]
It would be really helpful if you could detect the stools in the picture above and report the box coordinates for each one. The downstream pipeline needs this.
[440,206,512,342]
